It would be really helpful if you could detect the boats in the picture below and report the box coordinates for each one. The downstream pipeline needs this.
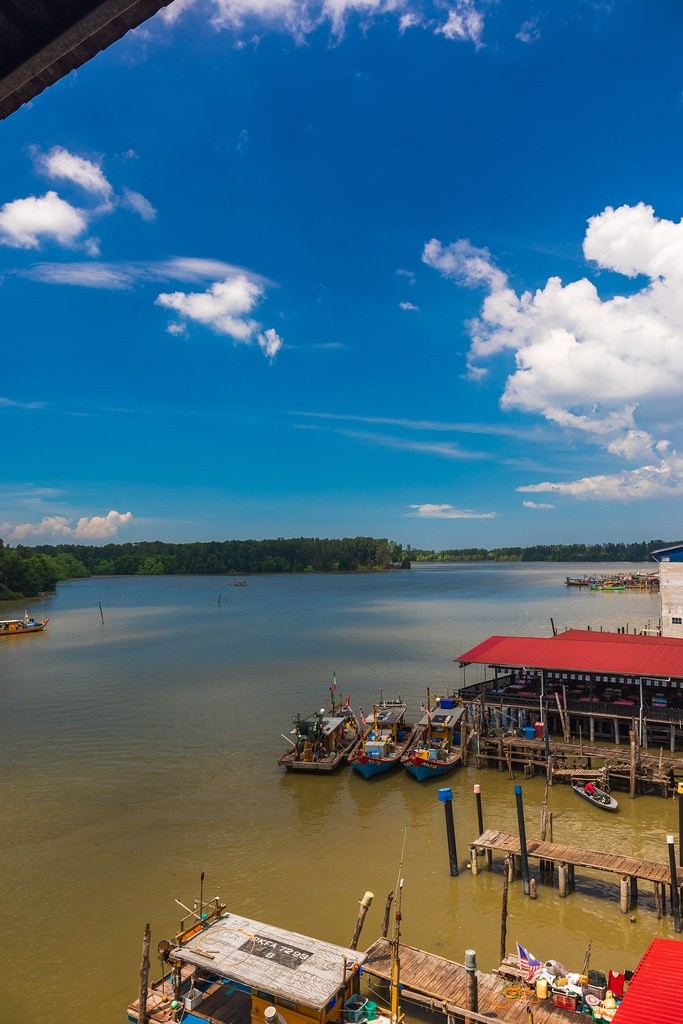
[275,668,363,772]
[347,687,420,779]
[400,687,474,781]
[0,610,49,635]
[572,784,618,810]
[587,583,625,590]
[234,580,248,587]
[124,868,406,1024]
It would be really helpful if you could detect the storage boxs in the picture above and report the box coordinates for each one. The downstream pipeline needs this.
[182,987,203,1011]
[525,727,535,740]
[343,994,368,1023]
[551,989,577,1010]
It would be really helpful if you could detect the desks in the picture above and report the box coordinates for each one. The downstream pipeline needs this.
[506,676,644,705]
[497,953,542,978]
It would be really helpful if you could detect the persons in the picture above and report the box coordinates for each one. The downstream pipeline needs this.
[585,781,599,796]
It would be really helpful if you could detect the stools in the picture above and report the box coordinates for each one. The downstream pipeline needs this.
[365,1001,377,1020]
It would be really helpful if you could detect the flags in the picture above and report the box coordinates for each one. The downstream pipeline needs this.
[519,943,542,982]
[420,702,427,712]
[359,708,367,727]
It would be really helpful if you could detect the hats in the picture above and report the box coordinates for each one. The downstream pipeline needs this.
[593,782,596,786]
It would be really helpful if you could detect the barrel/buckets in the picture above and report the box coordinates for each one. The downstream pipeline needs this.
[537,978,548,999]
[364,1001,377,1020]
